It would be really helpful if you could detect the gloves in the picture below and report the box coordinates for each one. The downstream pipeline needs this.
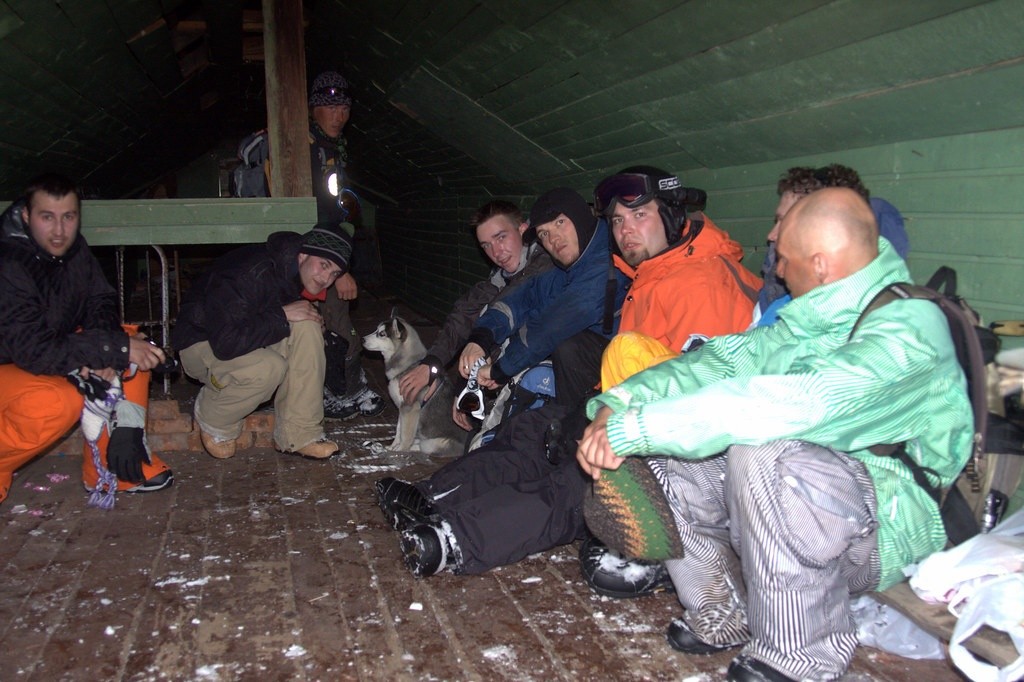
[64,373,110,402]
[106,427,151,483]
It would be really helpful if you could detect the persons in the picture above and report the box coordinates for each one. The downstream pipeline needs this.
[170,219,356,460]
[263,71,388,422]
[0,177,174,504]
[374,165,976,682]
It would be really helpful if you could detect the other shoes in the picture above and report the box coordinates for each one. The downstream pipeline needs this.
[578,542,675,597]
[376,477,457,578]
[274,437,339,460]
[323,386,387,422]
[81,422,174,492]
[667,616,739,654]
[199,428,235,458]
[727,653,794,682]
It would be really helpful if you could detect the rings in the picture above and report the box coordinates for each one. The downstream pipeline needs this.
[463,366,469,369]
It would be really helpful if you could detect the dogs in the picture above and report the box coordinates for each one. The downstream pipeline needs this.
[360,306,469,457]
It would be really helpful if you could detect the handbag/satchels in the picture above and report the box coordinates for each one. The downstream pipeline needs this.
[910,506,1024,682]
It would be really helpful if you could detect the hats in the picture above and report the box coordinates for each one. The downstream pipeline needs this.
[583,456,685,559]
[300,222,352,278]
[616,166,686,246]
[522,187,596,254]
[309,71,352,107]
[80,374,121,441]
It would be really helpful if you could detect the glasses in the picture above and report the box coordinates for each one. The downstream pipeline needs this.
[592,174,707,215]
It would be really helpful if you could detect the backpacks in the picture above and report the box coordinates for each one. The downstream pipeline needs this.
[847,266,1004,551]
[470,363,556,451]
[229,127,325,199]
